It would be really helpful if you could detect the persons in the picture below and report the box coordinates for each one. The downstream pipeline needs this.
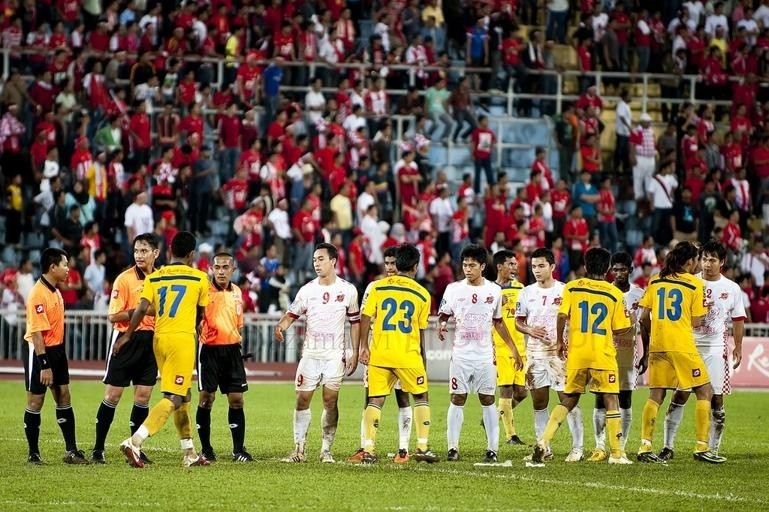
[0,2,768,465]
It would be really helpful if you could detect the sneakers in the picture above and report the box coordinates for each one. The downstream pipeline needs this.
[507,433,726,463]
[184,448,256,466]
[282,449,500,464]
[27,437,155,467]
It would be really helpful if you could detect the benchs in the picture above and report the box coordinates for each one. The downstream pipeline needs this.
[399,23,676,203]
